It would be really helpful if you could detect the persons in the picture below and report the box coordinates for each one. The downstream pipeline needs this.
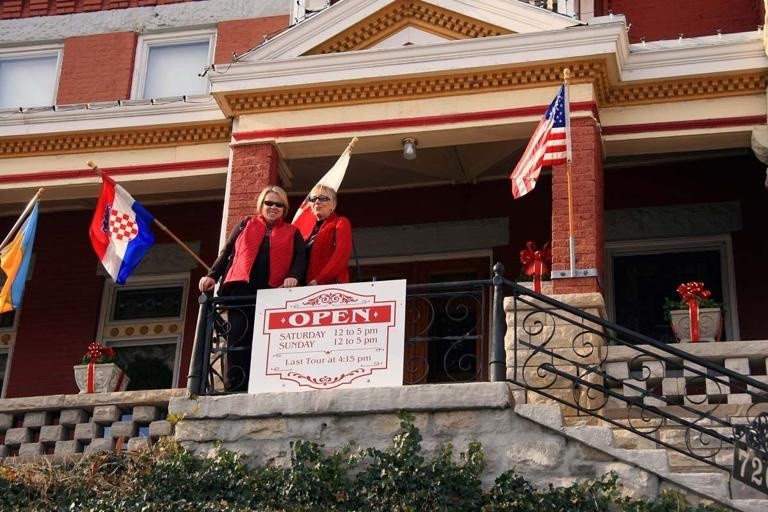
[198,185,308,394]
[297,184,353,285]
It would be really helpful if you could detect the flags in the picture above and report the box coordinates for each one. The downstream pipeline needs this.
[510,83,571,200]
[89,171,155,286]
[289,148,351,241]
[0,200,40,314]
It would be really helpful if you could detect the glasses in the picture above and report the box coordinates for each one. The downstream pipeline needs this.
[309,195,331,203]
[263,200,285,208]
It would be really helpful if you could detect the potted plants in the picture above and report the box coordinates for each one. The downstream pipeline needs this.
[515,239,554,294]
[71,340,134,394]
[663,280,727,344]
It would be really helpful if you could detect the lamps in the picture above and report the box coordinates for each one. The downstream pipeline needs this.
[401,137,419,162]
